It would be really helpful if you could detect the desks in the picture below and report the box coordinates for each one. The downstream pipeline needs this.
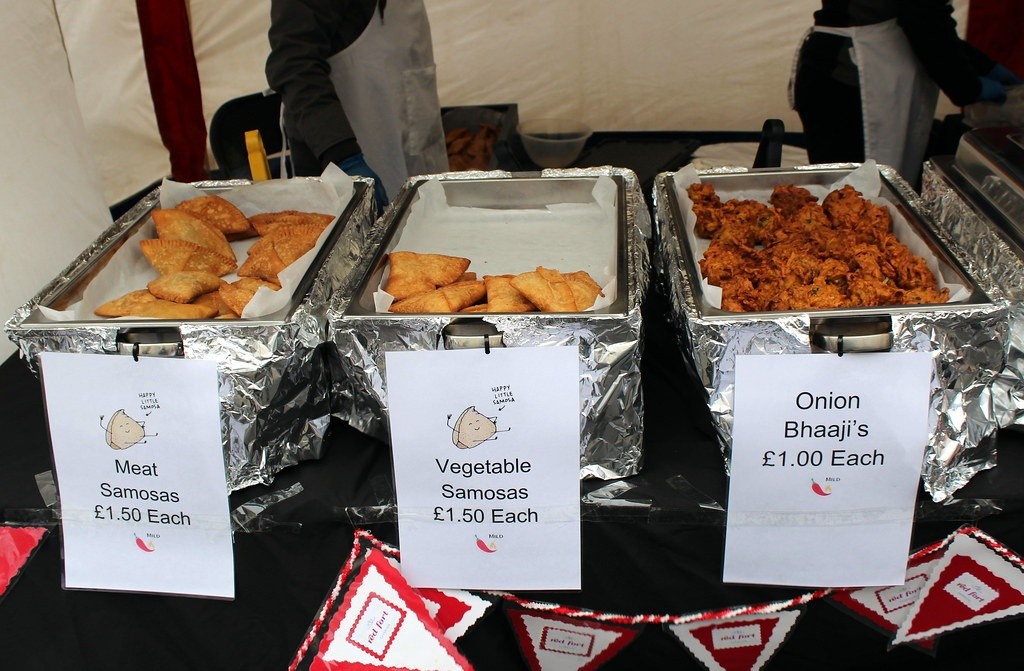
[0,288,1024,671]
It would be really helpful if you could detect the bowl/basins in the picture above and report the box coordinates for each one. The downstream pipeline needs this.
[517,117,591,168]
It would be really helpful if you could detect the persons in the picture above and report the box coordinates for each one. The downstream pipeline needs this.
[264,0,450,220]
[788,0,1016,187]
